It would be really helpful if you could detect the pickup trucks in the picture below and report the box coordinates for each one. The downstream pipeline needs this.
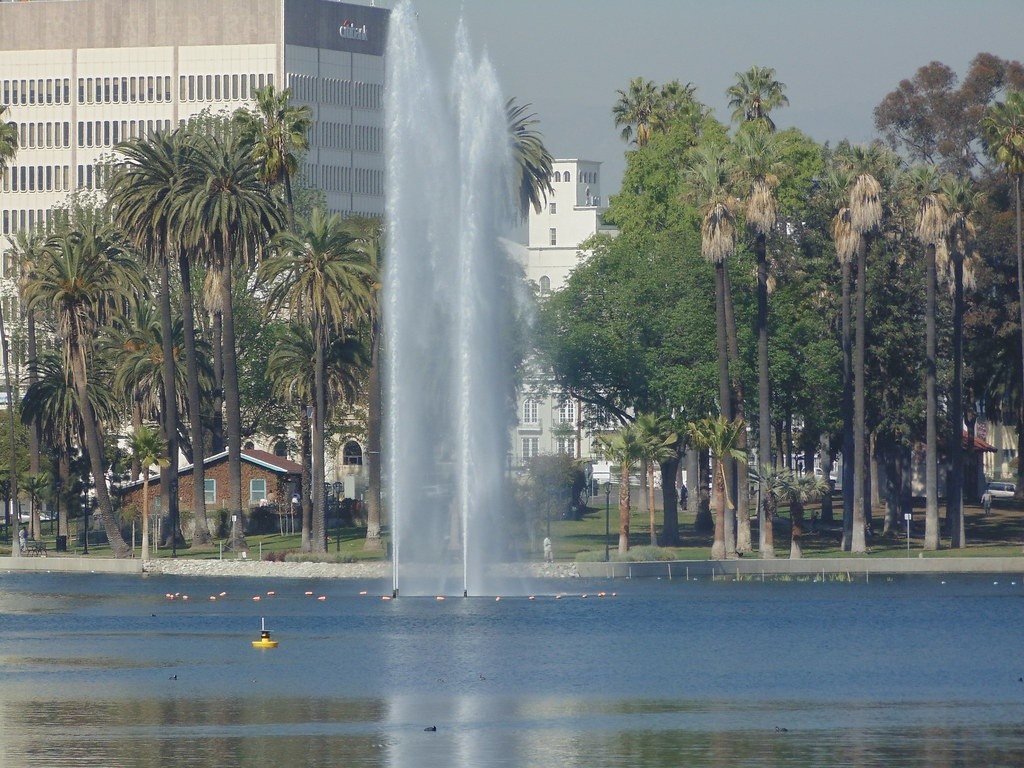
[798,466,838,490]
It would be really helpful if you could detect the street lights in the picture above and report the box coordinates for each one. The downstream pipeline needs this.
[2,480,11,541]
[543,474,553,537]
[55,480,62,551]
[169,478,179,558]
[505,452,514,479]
[324,481,333,553]
[82,484,89,554]
[28,476,37,537]
[332,481,342,551]
[603,481,614,561]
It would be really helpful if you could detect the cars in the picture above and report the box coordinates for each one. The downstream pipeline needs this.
[18,509,56,524]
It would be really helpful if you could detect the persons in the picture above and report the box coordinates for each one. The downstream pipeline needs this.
[981,490,992,515]
[19,527,29,552]
[866,522,873,551]
[810,512,820,537]
[543,533,554,563]
[268,489,282,506]
[356,500,362,518]
[681,485,687,510]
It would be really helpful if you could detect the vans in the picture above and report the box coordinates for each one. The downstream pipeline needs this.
[987,481,1016,498]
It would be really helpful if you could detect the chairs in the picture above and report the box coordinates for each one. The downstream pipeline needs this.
[26,541,49,558]
